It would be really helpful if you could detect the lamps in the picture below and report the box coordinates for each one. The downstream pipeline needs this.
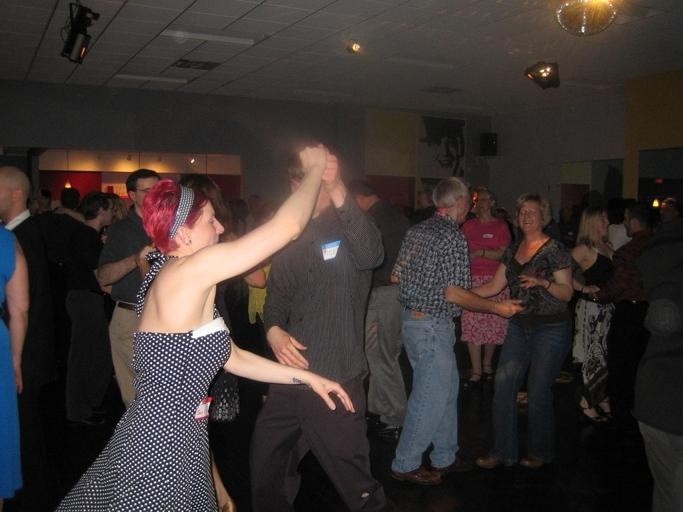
[57,0,101,64]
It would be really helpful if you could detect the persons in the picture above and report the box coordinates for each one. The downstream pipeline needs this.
[635,220,680,511]
[0,164,53,511]
[28,167,683,485]
[53,143,359,511]
[0,223,31,510]
[246,132,392,511]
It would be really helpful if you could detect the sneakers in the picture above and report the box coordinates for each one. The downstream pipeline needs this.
[67,407,106,425]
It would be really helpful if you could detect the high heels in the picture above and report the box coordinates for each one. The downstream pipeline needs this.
[598,399,616,419]
[577,402,609,424]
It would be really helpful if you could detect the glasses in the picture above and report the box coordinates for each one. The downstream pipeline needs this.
[136,187,150,194]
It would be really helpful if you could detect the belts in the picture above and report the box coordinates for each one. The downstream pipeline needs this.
[89,288,103,296]
[118,302,138,312]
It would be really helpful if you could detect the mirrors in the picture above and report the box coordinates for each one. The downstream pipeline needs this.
[637,148,683,228]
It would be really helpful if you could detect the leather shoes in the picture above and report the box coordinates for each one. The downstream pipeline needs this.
[463,372,482,391]
[476,455,502,468]
[392,467,442,485]
[430,467,450,473]
[483,365,495,380]
[367,415,400,441]
[519,453,545,467]
